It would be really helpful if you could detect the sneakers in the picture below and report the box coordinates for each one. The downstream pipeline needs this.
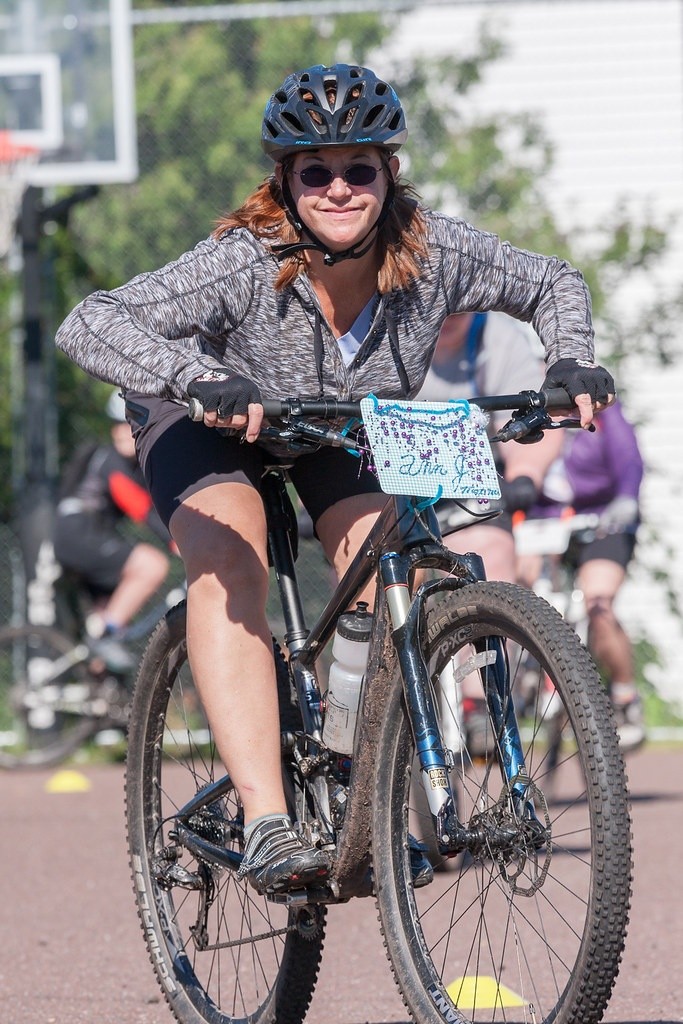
[236,819,331,895]
[325,773,434,888]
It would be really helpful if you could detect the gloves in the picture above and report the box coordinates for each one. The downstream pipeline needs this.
[509,477,538,510]
[599,494,637,533]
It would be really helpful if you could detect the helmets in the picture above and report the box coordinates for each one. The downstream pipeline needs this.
[105,387,127,422]
[259,64,406,161]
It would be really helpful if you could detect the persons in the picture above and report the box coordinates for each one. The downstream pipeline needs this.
[55,64,616,895]
[53,387,187,746]
[412,310,565,765]
[513,396,644,754]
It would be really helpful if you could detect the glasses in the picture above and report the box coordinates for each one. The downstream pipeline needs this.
[288,165,388,189]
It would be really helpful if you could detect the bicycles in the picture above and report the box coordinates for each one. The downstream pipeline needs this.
[501,507,630,803]
[120,396,632,1024]
[0,549,289,768]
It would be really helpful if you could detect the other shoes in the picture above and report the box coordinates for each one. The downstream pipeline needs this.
[465,711,495,756]
[612,698,644,750]
[80,617,133,673]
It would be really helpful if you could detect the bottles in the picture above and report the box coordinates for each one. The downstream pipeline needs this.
[323,601,373,756]
